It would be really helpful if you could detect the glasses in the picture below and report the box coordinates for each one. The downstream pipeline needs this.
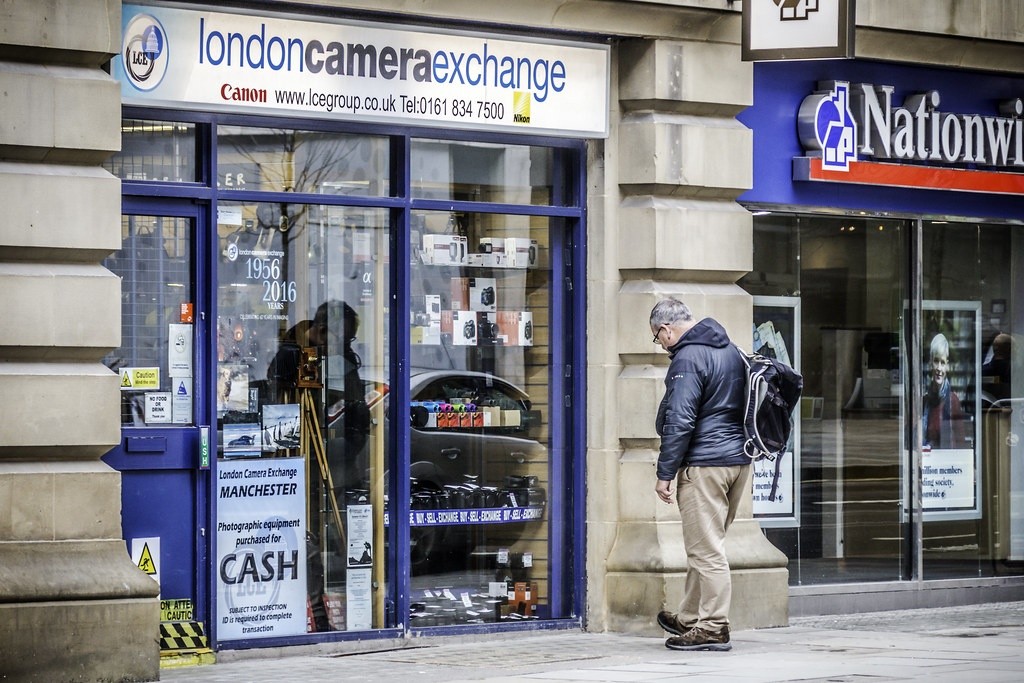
[321,324,356,344]
[653,323,670,344]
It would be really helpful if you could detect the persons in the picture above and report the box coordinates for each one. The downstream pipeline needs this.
[921,333,969,449]
[966,334,1024,414]
[265,298,366,635]
[649,296,755,651]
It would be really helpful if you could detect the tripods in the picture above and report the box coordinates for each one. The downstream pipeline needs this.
[298,389,347,550]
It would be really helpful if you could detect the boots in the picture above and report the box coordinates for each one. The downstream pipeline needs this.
[510,553,530,590]
[495,554,514,591]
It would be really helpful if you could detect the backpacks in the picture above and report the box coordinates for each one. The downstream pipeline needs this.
[729,342,803,461]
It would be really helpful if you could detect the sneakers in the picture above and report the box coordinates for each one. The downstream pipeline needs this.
[665,627,732,651]
[657,611,693,636]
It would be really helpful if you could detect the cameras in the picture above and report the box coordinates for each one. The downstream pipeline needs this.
[293,346,323,388]
[477,312,498,338]
[524,320,531,339]
[414,310,431,328]
[481,287,495,306]
[409,474,545,510]
[464,320,475,339]
[478,243,492,253]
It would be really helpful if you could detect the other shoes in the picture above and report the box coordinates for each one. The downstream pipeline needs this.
[314,616,331,631]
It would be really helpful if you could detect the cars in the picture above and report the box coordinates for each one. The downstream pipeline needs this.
[301,363,548,577]
